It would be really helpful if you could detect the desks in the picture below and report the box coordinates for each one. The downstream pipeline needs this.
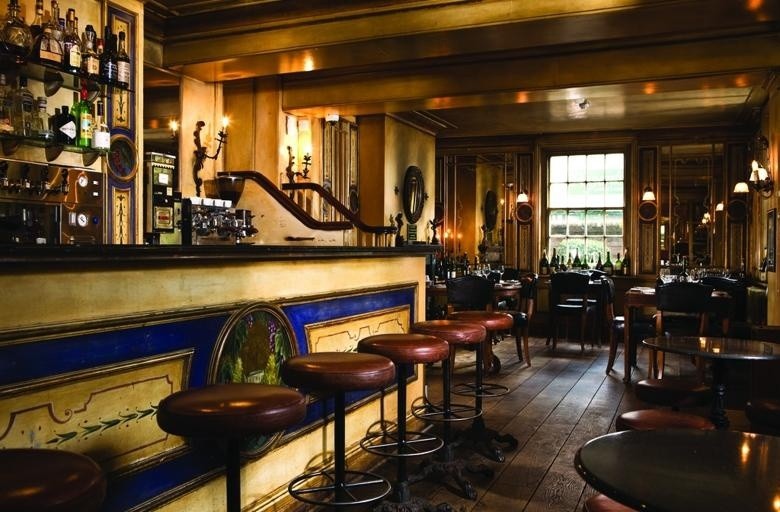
[575,426,779,512]
[425,275,523,311]
[644,336,779,428]
[622,283,734,384]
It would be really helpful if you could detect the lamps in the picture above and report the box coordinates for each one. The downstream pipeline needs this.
[324,114,340,127]
[641,186,655,201]
[750,160,772,199]
[287,137,312,184]
[194,117,229,196]
[716,200,725,212]
[733,181,750,195]
[578,98,592,110]
[515,190,529,204]
[701,211,710,226]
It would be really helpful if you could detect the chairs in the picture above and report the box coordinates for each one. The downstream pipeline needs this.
[516,274,538,323]
[646,284,708,378]
[600,276,659,381]
[446,278,532,373]
[501,267,518,278]
[701,278,746,337]
[548,272,590,352]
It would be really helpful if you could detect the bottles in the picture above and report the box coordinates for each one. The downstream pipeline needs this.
[438,259,445,280]
[1,1,33,61]
[615,253,622,275]
[77,88,91,148]
[34,21,62,68]
[33,97,54,140]
[61,9,82,75]
[581,254,589,271]
[92,100,110,152]
[567,253,572,268]
[550,249,558,274]
[462,258,467,276]
[603,252,613,276]
[13,74,34,137]
[115,32,131,86]
[0,73,12,134]
[595,253,603,271]
[100,27,117,80]
[27,0,41,42]
[81,24,100,77]
[572,248,581,271]
[450,260,456,279]
[444,260,451,280]
[622,249,631,276]
[58,105,76,146]
[47,0,62,44]
[559,256,566,271]
[540,248,548,274]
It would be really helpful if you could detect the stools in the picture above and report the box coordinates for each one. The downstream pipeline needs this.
[742,396,780,431]
[353,332,448,509]
[1,449,107,512]
[410,320,483,459]
[449,309,517,463]
[585,494,643,512]
[282,353,395,509]
[157,380,305,512]
[635,377,711,410]
[613,409,715,434]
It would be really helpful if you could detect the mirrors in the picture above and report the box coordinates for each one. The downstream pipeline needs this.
[447,152,509,268]
[659,138,727,270]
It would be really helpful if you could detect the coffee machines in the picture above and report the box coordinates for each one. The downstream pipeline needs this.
[184,171,258,245]
